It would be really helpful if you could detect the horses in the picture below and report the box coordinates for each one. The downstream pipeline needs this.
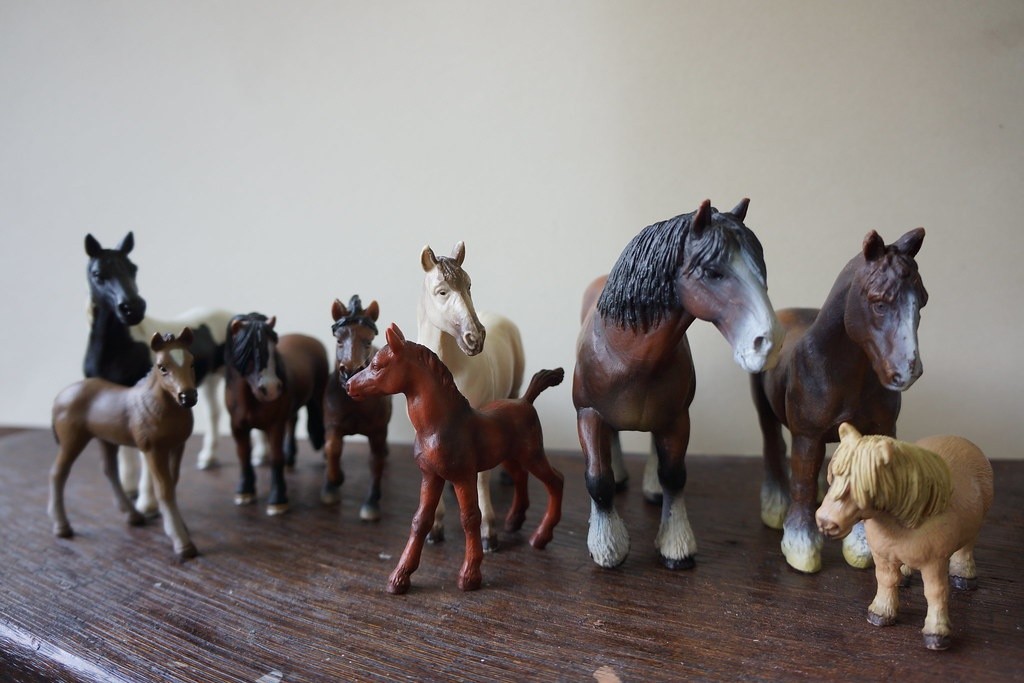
[570,197,782,574]
[47,231,236,566]
[225,313,330,518]
[748,225,997,653]
[320,236,564,597]
